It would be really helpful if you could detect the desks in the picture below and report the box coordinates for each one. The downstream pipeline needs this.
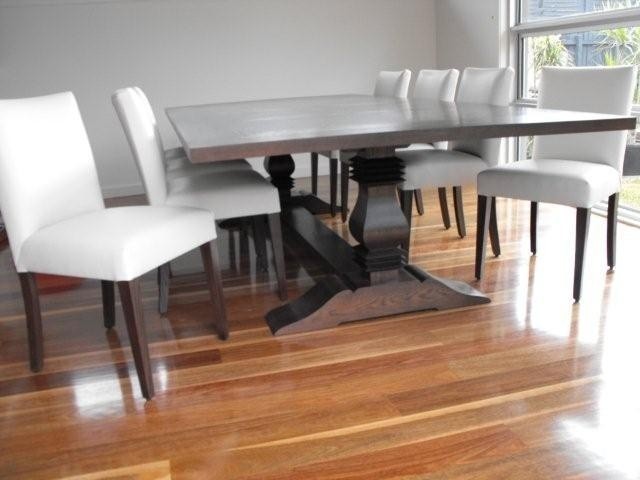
[165,94,636,338]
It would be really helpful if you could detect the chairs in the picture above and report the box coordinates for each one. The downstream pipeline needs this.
[310,69,411,218]
[476,66,639,300]
[395,67,521,257]
[1,92,229,400]
[339,69,459,229]
